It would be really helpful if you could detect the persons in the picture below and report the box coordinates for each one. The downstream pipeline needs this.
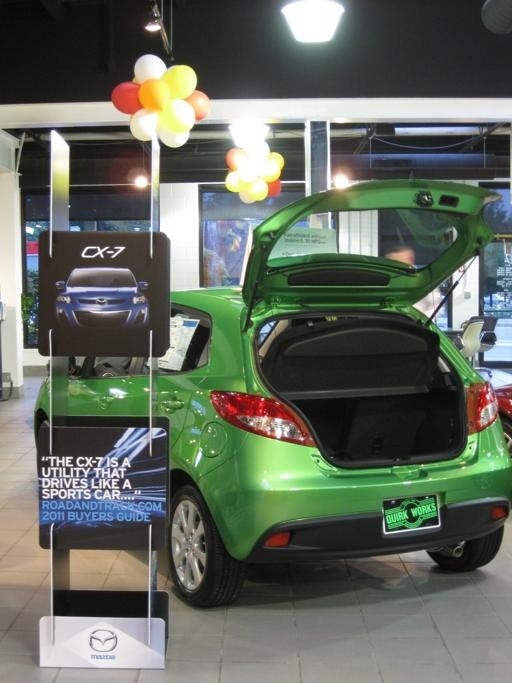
[383,245,445,327]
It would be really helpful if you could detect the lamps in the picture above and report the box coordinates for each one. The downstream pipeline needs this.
[281,0,344,43]
[144,16,162,33]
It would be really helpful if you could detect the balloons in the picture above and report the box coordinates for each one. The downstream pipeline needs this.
[222,142,283,204]
[110,52,211,147]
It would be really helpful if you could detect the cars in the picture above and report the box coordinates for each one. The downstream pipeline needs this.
[52,266,150,330]
[477,366,512,459]
[30,178,512,612]
